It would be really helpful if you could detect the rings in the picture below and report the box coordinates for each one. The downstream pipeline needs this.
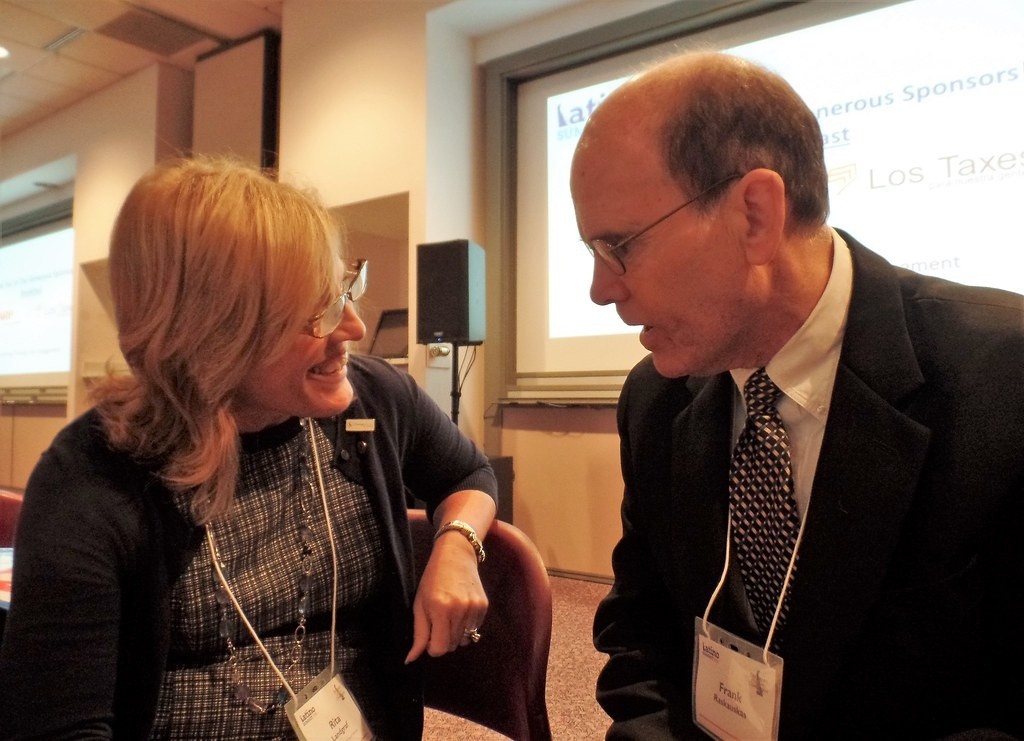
[464,626,482,643]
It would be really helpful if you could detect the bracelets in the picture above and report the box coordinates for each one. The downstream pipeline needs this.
[429,519,486,566]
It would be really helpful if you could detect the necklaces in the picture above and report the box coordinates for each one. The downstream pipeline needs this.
[191,416,318,717]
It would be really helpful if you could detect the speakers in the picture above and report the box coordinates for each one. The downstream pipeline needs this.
[416,240,486,346]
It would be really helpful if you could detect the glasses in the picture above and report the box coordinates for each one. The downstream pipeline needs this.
[296,259,369,337]
[582,172,745,274]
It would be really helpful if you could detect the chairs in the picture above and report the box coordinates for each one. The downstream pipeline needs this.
[405,508,556,740]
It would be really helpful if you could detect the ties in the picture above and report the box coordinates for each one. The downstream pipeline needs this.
[729,373,803,641]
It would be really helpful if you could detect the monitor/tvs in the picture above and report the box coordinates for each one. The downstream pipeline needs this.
[367,308,408,359]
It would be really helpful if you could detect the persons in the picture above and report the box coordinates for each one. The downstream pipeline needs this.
[1,154,498,739]
[566,50,1024,740]
